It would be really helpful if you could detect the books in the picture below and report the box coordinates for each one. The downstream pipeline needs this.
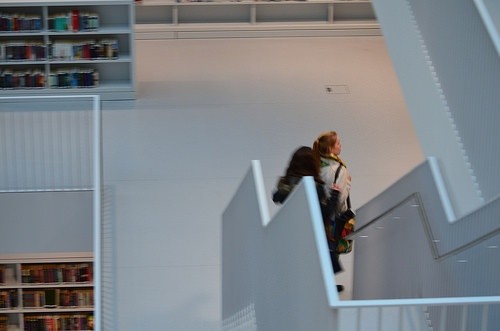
[0,259,95,330]
[0,6,119,92]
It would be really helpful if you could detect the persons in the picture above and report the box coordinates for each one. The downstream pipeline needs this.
[271,146,342,226]
[311,131,355,293]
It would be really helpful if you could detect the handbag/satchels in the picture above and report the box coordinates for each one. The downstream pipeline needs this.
[334,209,356,254]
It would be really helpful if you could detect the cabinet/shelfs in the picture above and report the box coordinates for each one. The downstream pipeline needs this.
[0,1,134,101]
[0,253,94,331]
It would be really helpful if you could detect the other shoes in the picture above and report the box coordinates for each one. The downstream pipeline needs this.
[336,284,344,292]
